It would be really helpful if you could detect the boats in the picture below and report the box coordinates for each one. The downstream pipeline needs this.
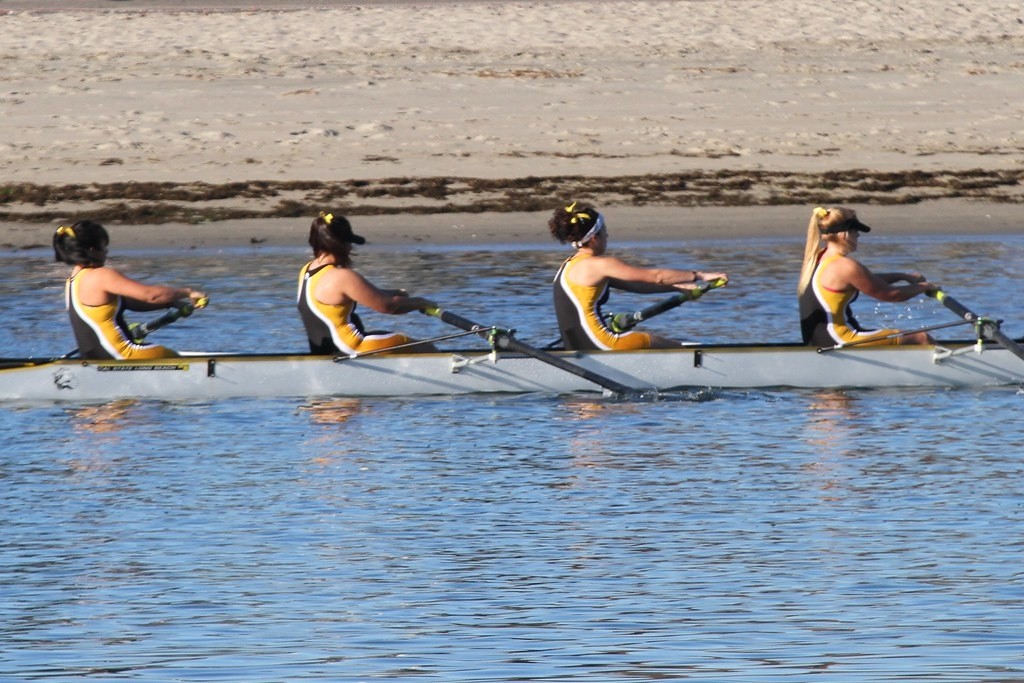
[0,326,1024,400]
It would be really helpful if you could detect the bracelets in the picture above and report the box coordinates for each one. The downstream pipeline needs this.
[692,271,699,283]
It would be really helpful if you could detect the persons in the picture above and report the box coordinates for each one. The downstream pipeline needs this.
[52,218,207,362]
[796,206,943,349]
[547,199,729,350]
[296,210,441,357]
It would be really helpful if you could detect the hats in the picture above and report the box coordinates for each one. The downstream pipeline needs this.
[819,214,871,233]
[309,223,366,244]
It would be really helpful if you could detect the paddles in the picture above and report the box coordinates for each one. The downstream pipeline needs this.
[924,286,1024,361]
[127,297,210,345]
[420,307,635,395]
[608,278,728,335]
